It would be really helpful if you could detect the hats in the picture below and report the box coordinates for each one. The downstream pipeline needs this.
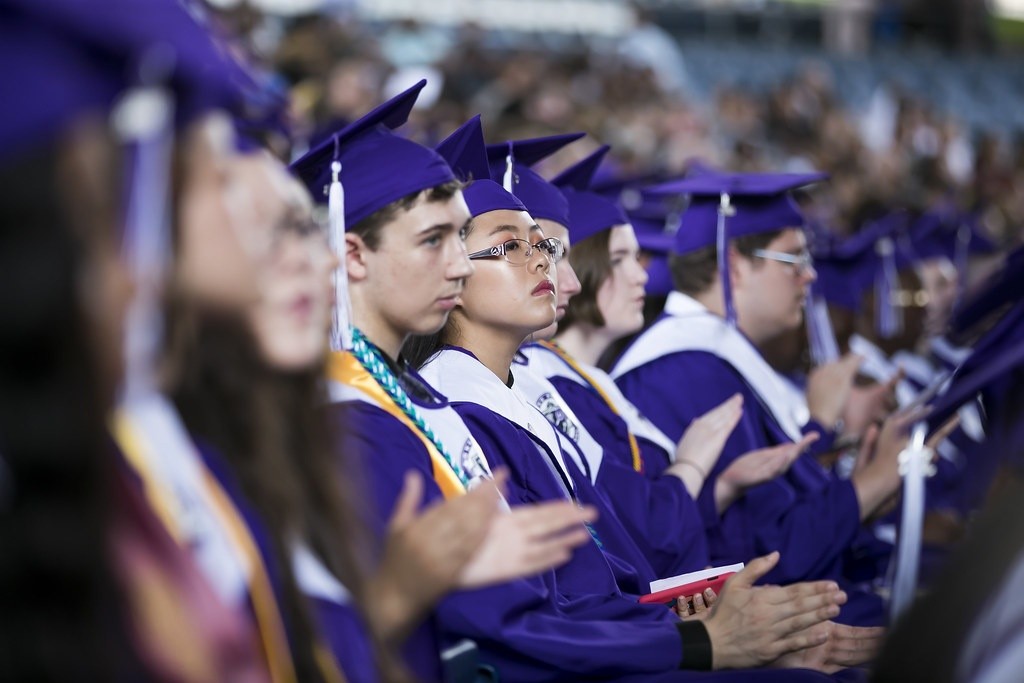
[0,0,292,286]
[592,162,1000,368]
[287,80,456,354]
[546,146,631,248]
[435,112,531,219]
[487,132,587,232]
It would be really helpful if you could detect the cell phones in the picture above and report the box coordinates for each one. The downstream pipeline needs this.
[638,571,738,617]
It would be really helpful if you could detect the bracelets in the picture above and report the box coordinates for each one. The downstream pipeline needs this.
[674,460,705,481]
[674,620,713,672]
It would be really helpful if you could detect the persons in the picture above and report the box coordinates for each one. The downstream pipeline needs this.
[0,0,1024,683]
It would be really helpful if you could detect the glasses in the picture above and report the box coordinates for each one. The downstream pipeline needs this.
[467,238,567,265]
[749,250,813,274]
